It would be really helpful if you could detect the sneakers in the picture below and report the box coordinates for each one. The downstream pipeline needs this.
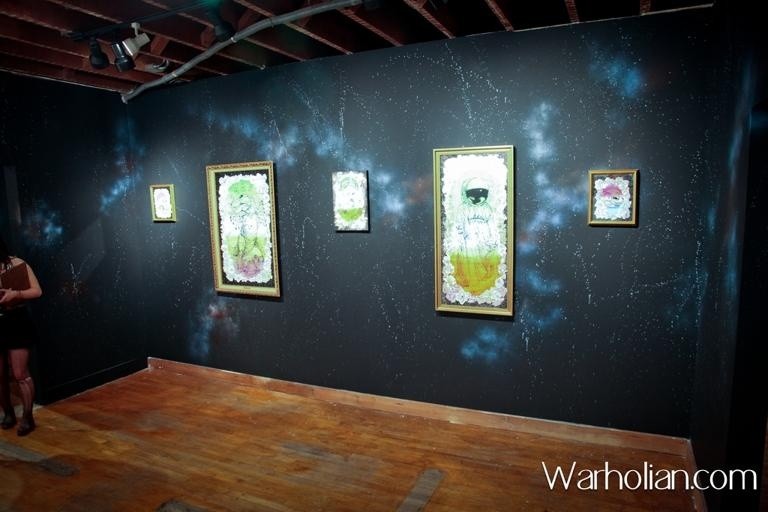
[17,418,34,435]
[1,414,16,429]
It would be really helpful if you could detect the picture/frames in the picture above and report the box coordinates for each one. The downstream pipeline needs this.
[149,184,177,222]
[205,160,281,299]
[432,146,515,317]
[586,169,639,227]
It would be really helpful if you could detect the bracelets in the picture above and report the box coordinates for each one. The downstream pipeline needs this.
[19,290,21,299]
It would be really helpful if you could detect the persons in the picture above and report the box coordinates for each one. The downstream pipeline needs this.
[0,237,42,436]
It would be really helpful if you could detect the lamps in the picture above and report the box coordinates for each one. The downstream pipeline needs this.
[86,24,152,73]
